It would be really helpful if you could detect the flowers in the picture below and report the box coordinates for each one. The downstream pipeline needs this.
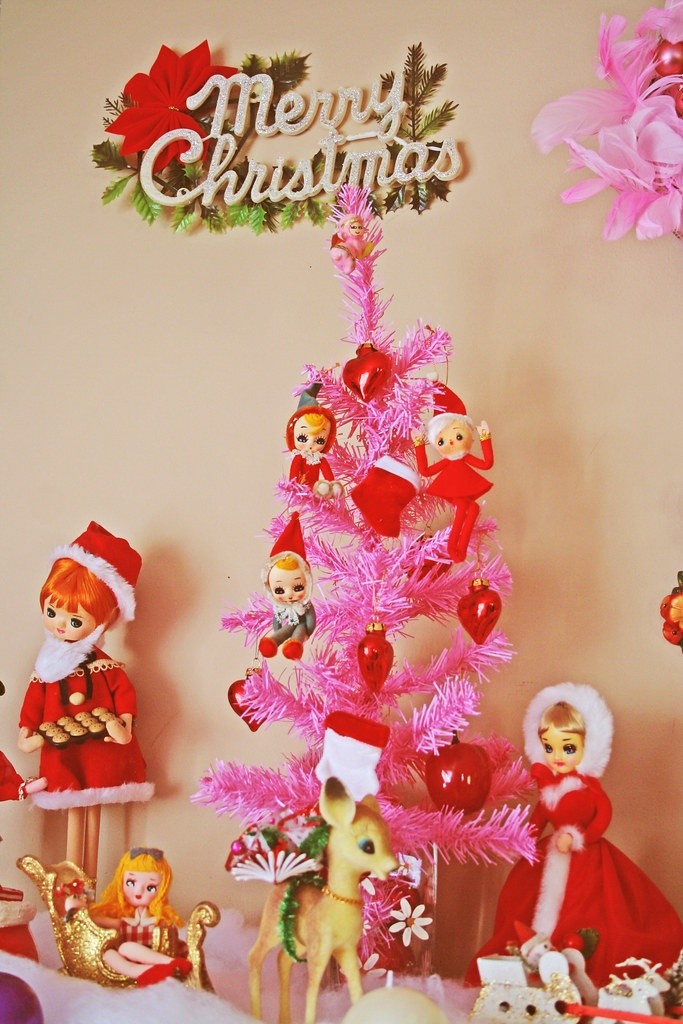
[528,2,682,244]
[104,40,239,172]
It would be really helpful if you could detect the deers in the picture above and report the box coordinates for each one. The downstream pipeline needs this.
[246,773,400,1023]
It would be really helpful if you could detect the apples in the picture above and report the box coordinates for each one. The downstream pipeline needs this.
[422,728,491,816]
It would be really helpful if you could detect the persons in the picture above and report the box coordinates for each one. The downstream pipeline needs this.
[0,402,682,1017]
